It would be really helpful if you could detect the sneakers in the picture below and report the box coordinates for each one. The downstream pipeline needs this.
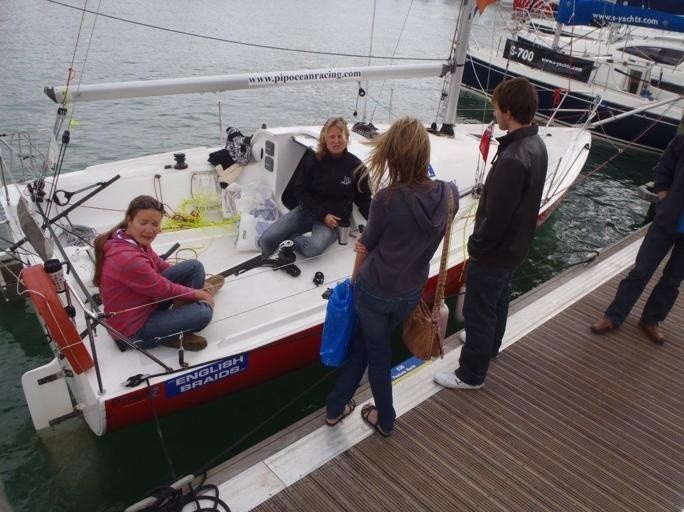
[432,370,485,389]
[460,330,466,344]
[202,275,224,297]
[164,333,207,351]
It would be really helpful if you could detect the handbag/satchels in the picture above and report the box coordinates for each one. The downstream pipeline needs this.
[402,299,444,361]
[319,278,358,368]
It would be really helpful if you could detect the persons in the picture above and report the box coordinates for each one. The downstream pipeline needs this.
[591,115,683,344]
[434,76,548,390]
[94,195,225,351]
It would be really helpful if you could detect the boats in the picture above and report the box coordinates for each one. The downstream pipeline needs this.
[0,121,596,439]
[458,0,684,152]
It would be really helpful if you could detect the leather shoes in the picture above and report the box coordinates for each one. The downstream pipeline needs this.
[591,317,617,334]
[638,319,666,345]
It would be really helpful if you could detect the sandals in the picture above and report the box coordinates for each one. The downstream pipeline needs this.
[325,398,355,426]
[361,403,393,437]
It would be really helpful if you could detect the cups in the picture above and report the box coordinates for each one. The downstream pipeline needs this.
[43,258,71,294]
[337,221,350,246]
